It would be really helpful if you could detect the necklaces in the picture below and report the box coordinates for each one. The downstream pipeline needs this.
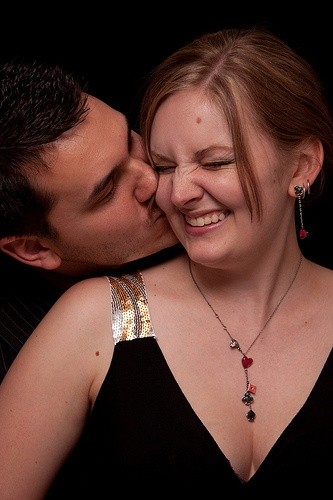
[188,251,305,423]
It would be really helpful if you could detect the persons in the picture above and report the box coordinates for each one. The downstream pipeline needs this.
[0,28,333,500]
